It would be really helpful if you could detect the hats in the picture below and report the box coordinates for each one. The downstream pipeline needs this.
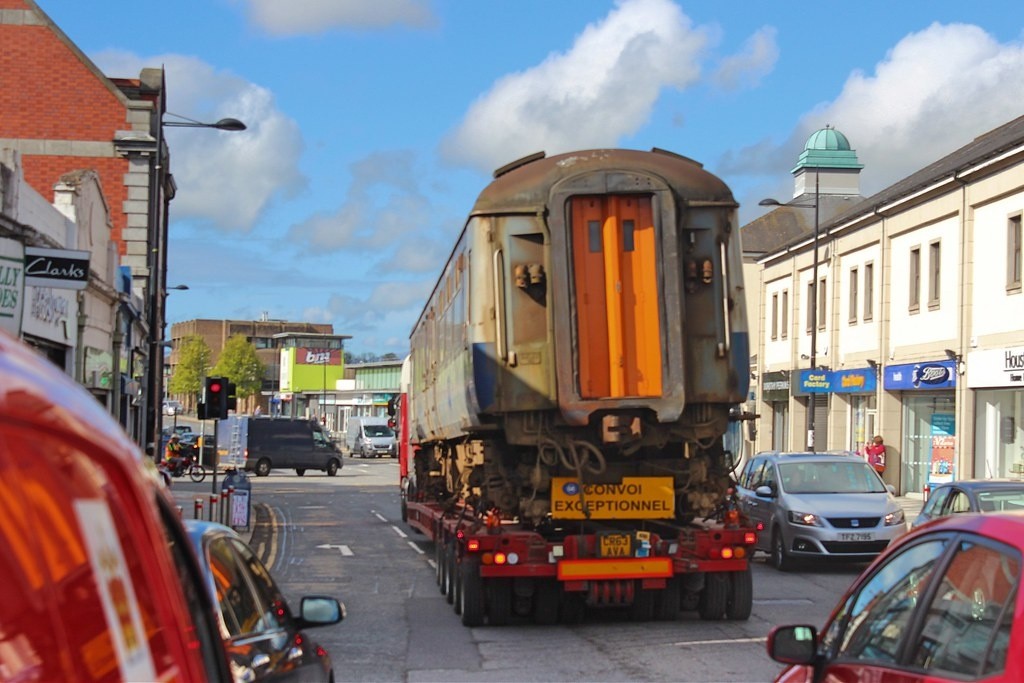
[171,433,181,438]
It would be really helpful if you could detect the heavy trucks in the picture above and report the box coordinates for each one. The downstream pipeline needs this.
[386,147,757,630]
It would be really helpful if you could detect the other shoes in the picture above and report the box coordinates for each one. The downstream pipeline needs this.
[174,472,183,476]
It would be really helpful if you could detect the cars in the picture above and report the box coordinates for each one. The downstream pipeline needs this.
[764,509,1024,682]
[179,516,347,683]
[162,425,215,470]
[912,480,1024,530]
[731,449,908,572]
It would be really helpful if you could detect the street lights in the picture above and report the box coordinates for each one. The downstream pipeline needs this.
[323,344,344,426]
[757,163,820,453]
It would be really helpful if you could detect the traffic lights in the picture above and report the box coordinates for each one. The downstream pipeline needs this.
[196,402,206,420]
[228,383,239,414]
[206,377,228,421]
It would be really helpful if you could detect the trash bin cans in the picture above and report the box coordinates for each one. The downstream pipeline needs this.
[222,471,251,532]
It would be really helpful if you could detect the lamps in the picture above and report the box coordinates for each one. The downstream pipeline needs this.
[780,370,790,377]
[866,359,881,369]
[820,365,832,371]
[750,373,759,380]
[945,349,963,362]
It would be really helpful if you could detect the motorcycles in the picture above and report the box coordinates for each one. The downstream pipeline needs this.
[161,447,206,483]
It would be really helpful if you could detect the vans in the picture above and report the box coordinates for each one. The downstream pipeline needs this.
[347,416,397,460]
[162,401,183,417]
[216,414,345,478]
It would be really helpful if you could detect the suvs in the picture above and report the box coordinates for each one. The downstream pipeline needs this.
[0,328,233,682]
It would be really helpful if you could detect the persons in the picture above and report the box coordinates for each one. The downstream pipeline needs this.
[165,433,189,464]
[865,435,886,478]
